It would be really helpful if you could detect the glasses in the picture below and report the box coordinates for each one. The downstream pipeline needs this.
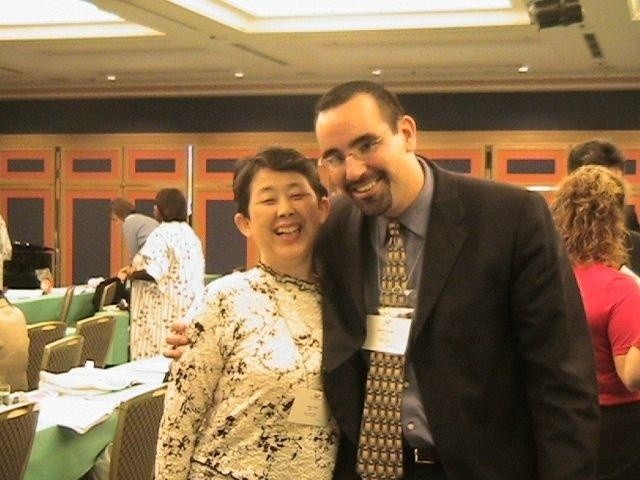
[317,127,390,170]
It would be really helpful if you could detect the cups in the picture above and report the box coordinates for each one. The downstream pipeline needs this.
[0,383,11,411]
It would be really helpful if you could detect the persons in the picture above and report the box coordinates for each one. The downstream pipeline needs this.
[107,198,161,267]
[548,166,640,480]
[163,81,603,480]
[566,139,640,283]
[0,288,29,392]
[123,189,205,362]
[154,145,340,480]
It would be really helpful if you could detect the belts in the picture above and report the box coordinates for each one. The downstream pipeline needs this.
[405,447,440,466]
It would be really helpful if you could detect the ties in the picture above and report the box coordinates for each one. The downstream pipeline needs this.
[356,219,410,480]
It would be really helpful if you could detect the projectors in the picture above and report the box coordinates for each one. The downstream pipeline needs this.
[523,0,584,30]
